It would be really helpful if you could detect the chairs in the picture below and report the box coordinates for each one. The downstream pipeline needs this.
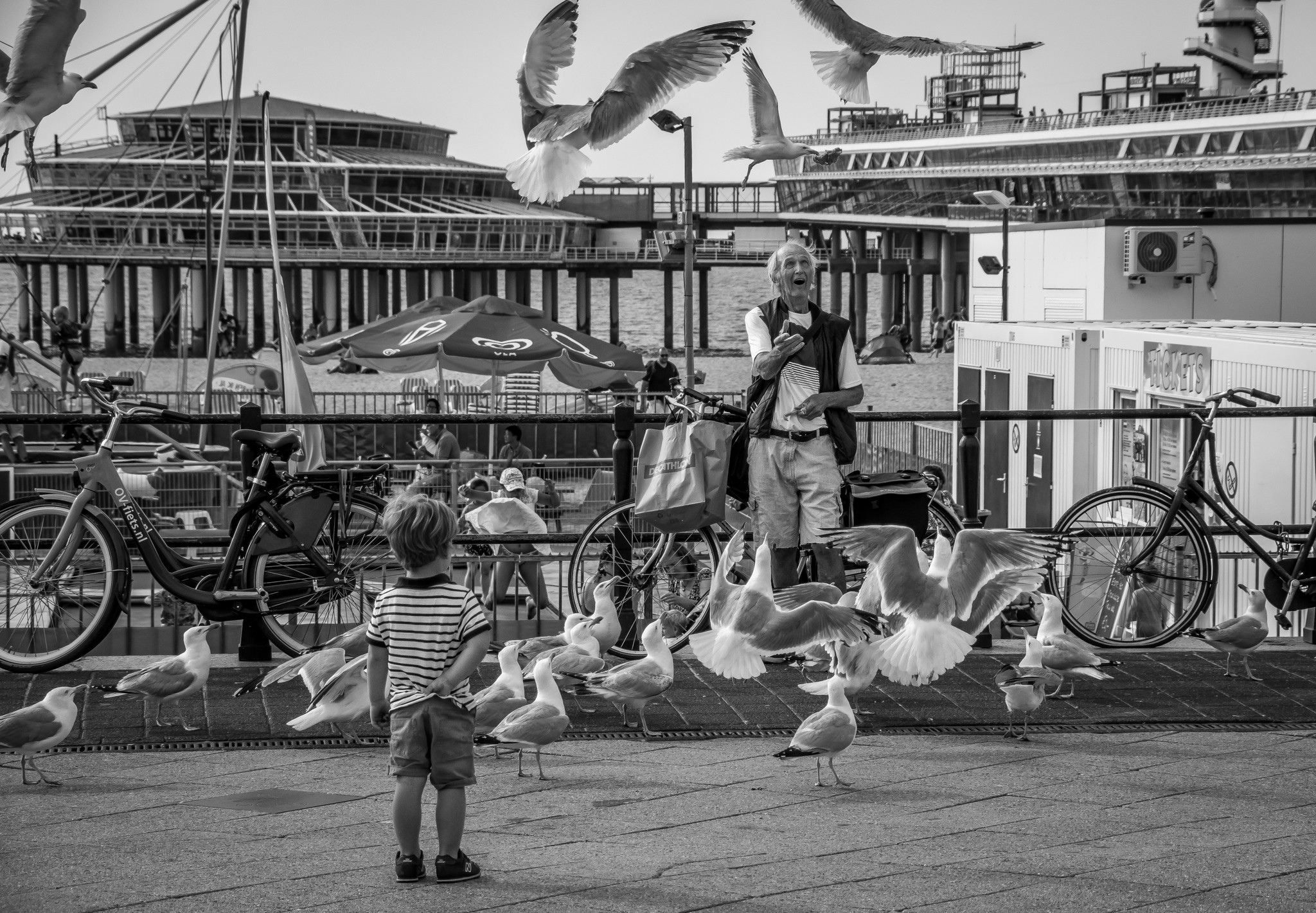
[27,371,151,412]
[397,373,505,414]
[208,387,280,414]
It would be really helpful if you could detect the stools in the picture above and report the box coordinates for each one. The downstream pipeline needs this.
[175,510,216,559]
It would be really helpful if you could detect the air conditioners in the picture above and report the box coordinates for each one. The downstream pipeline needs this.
[1123,227,1207,276]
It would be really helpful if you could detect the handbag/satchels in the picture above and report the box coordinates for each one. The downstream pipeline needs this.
[838,469,931,547]
[634,407,734,534]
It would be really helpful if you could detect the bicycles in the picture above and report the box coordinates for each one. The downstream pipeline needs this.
[0,376,412,676]
[566,375,968,662]
[1043,385,1313,649]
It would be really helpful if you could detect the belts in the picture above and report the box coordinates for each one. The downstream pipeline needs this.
[769,427,829,442]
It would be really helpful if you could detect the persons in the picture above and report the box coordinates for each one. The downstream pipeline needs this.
[365,495,493,883]
[833,107,1065,144]
[1204,31,1298,112]
[615,339,683,413]
[405,398,562,621]
[1124,563,1168,638]
[921,465,966,567]
[143,589,196,627]
[743,237,864,665]
[1019,178,1243,219]
[40,306,93,401]
[217,307,240,359]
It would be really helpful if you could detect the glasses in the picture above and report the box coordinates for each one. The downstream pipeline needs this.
[660,354,668,357]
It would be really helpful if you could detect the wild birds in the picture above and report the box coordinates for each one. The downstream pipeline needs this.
[726,47,818,190]
[504,0,756,206]
[91,622,222,731]
[0,0,95,183]
[1177,583,1269,680]
[230,621,390,745]
[0,684,90,788]
[688,518,1118,789]
[472,574,675,781]
[795,1,1043,106]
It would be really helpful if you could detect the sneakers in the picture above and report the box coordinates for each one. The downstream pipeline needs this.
[436,847,481,883]
[395,849,426,882]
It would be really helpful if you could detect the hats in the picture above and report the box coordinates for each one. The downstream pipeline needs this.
[500,468,525,492]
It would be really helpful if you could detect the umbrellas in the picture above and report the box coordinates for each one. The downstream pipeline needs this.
[293,292,648,477]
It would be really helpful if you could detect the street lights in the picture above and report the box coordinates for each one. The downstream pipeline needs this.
[649,110,695,414]
[973,189,1017,321]
